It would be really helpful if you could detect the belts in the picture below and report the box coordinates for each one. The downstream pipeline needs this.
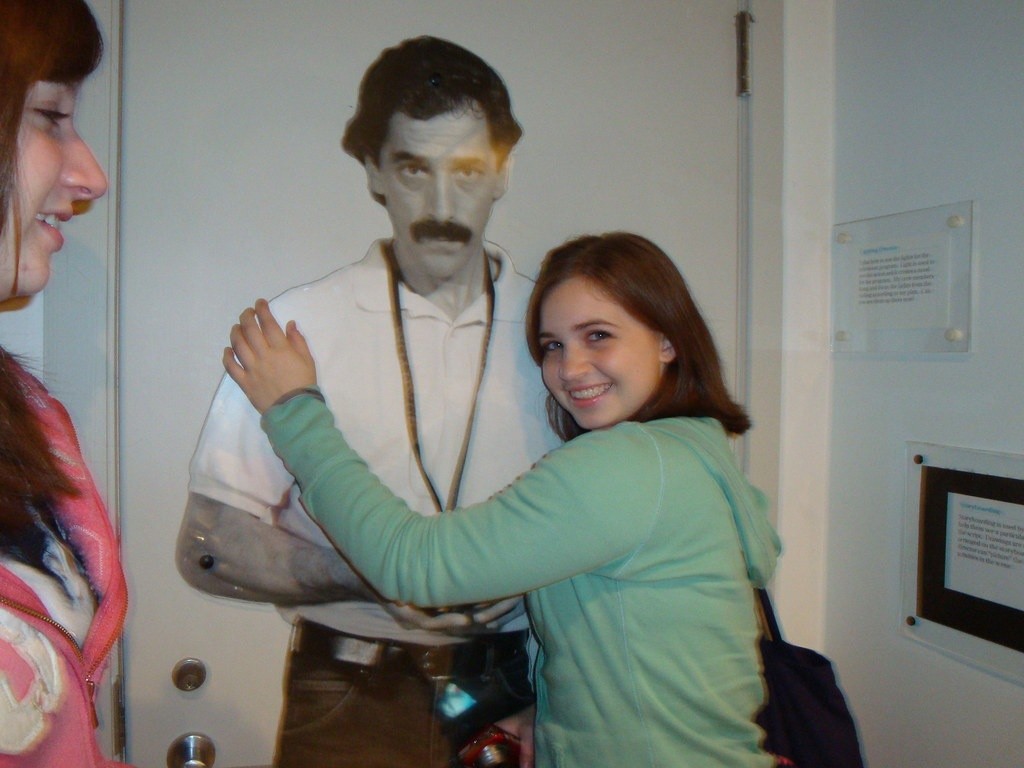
[298,623,531,682]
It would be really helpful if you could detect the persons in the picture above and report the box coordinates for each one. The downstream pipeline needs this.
[174,37,571,768]
[222,231,783,768]
[0,1,130,768]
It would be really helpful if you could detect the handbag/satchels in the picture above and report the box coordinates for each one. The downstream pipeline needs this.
[752,639,864,768]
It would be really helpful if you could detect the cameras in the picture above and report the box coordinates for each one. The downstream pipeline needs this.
[431,632,535,757]
